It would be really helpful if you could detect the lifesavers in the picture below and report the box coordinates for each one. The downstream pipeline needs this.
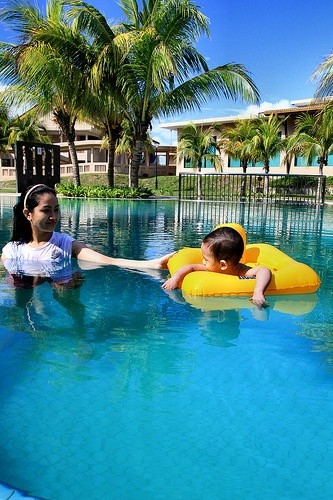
[182,294,319,316]
[166,221,322,298]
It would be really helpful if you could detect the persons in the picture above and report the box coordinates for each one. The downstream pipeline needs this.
[0,184,181,326]
[162,288,271,347]
[161,228,272,310]
